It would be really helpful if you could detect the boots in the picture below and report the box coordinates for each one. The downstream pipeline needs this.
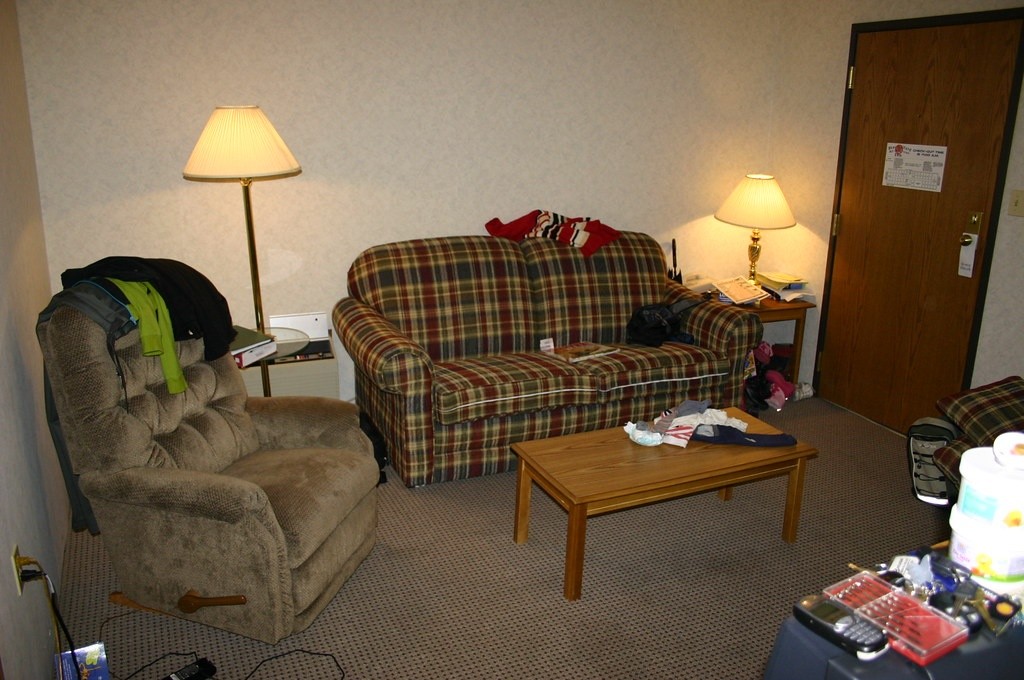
[765,370,794,412]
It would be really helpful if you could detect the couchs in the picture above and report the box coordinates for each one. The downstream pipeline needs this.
[37,277,380,644]
[932,435,980,491]
[332,231,763,491]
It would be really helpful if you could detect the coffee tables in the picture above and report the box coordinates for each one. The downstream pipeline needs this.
[510,406,818,600]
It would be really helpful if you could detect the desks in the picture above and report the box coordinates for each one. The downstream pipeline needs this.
[246,327,309,362]
[761,547,1024,680]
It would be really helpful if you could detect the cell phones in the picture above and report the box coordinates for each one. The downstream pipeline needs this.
[794,595,891,662]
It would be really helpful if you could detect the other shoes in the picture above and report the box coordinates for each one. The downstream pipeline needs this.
[793,381,814,402]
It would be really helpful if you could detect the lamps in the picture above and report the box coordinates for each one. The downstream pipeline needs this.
[714,173,796,306]
[182,105,303,398]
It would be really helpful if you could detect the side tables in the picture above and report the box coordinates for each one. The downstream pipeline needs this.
[706,294,817,387]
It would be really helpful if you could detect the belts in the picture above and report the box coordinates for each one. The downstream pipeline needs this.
[107,310,139,414]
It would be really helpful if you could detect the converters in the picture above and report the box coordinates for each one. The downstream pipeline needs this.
[161,657,217,680]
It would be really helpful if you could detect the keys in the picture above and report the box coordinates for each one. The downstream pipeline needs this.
[950,581,996,631]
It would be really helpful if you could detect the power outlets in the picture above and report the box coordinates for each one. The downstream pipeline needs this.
[10,544,25,597]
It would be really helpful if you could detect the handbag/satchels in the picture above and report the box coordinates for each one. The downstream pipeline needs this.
[626,291,712,348]
[906,416,961,507]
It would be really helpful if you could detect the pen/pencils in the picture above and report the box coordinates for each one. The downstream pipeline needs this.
[848,562,902,593]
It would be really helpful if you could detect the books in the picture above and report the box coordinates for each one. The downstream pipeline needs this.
[757,273,815,302]
[544,341,621,364]
[228,325,277,368]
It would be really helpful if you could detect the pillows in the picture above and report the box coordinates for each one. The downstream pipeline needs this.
[936,375,1024,448]
